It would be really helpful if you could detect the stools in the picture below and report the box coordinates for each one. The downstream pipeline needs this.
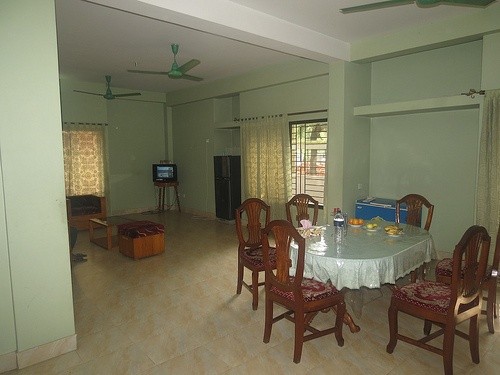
[118,220,165,258]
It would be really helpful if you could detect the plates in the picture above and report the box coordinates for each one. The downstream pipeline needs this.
[388,234,401,237]
[368,229,378,231]
[348,224,362,227]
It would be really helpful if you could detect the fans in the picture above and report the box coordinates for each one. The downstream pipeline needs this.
[339,1,495,14]
[73,75,141,100]
[127,43,206,83]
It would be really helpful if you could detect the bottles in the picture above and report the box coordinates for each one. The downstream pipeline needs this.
[333,207,347,246]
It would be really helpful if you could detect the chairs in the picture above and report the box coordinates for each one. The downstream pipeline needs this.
[262,221,360,363]
[384,226,490,375]
[394,192,435,231]
[422,226,500,340]
[237,198,294,310]
[285,194,319,227]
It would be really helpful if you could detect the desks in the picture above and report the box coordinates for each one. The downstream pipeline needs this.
[154,182,180,213]
[355,196,407,224]
[287,220,437,320]
[88,215,133,249]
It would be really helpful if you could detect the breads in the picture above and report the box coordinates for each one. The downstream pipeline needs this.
[385,225,398,235]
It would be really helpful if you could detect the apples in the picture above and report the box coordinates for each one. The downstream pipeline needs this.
[348,218,364,225]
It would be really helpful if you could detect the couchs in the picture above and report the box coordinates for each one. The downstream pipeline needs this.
[67,194,107,230]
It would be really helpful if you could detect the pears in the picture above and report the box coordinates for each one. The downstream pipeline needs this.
[366,223,372,229]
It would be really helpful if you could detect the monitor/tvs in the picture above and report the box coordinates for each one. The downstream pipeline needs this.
[152,164,177,183]
